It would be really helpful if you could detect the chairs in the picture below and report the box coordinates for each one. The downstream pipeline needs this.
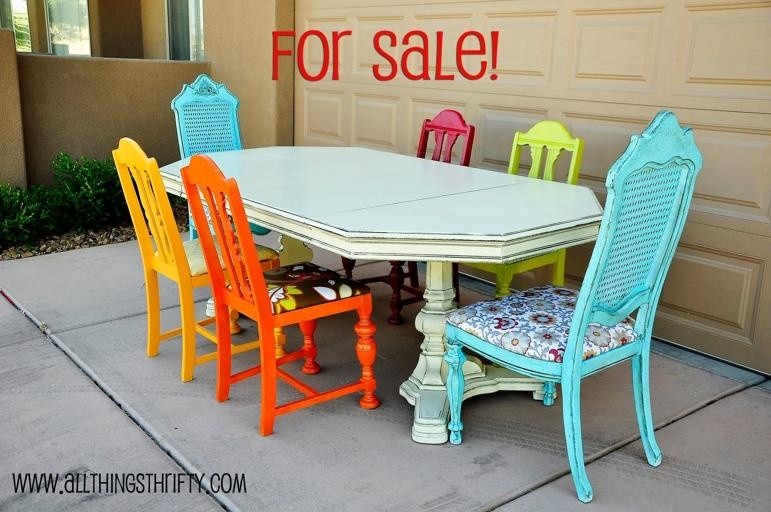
[341,109,476,323]
[169,73,272,239]
[459,120,586,300]
[112,137,289,383]
[444,109,704,504]
[180,153,381,436]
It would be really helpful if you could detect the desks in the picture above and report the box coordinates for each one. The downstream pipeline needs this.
[154,145,605,445]
